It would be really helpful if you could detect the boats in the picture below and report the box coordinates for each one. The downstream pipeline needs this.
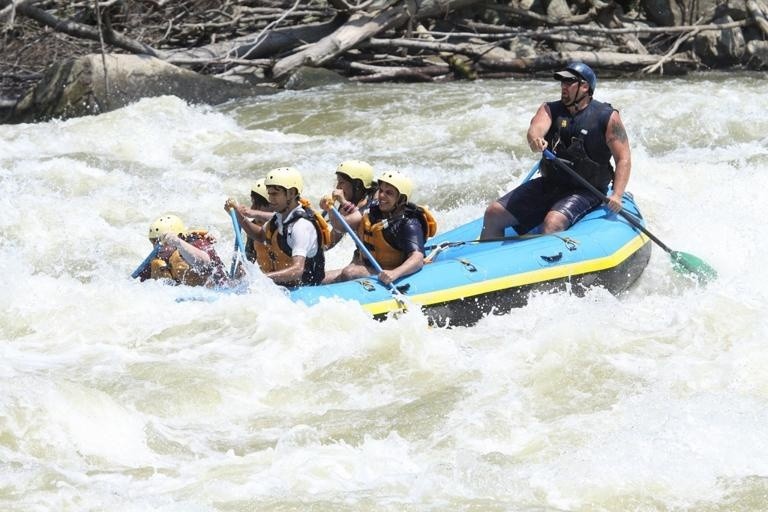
[177,190,653,335]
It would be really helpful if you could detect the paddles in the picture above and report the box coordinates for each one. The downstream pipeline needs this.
[227,205,261,279]
[325,203,422,314]
[542,148,716,283]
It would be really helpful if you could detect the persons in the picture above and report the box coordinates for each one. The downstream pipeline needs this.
[317,172,439,285]
[142,212,229,291]
[223,168,331,287]
[478,62,633,243]
[322,161,379,285]
[230,177,276,283]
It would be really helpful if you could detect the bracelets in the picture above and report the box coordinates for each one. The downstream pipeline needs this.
[341,202,357,214]
[240,215,247,225]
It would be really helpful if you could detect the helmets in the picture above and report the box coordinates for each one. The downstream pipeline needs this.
[148,214,188,242]
[251,183,271,204]
[335,159,374,190]
[552,61,597,97]
[376,170,413,206]
[264,166,304,201]
